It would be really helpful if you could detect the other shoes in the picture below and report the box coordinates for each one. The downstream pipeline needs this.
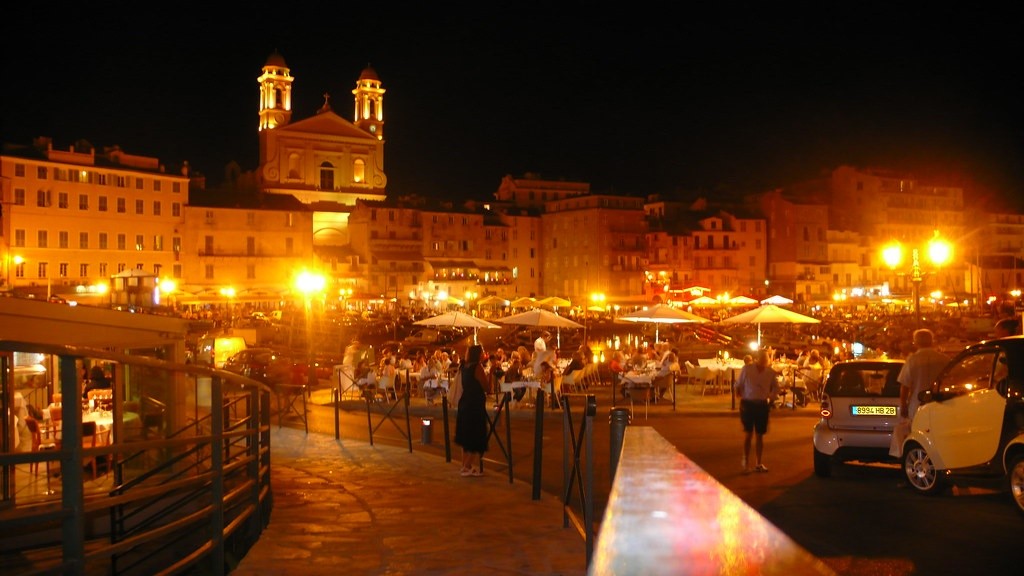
[461,467,484,478]
[756,464,768,472]
[802,397,810,407]
[897,483,908,488]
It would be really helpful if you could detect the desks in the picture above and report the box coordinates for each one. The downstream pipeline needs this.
[369,364,406,398]
[618,369,657,401]
[64,409,144,479]
[772,361,820,400]
[698,356,744,391]
[511,379,544,406]
[772,376,806,406]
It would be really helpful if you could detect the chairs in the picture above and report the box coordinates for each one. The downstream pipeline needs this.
[334,345,828,420]
[881,369,900,395]
[841,371,871,395]
[24,386,166,485]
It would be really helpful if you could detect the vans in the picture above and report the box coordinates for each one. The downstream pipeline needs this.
[405,329,440,342]
[183,336,246,367]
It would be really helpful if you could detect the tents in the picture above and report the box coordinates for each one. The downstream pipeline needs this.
[108,268,157,307]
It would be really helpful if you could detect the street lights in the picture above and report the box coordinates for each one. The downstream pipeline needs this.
[298,270,324,385]
[583,293,605,347]
[882,236,951,330]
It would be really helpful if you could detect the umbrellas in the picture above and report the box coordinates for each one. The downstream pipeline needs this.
[893,300,909,308]
[614,296,822,350]
[947,302,968,311]
[412,295,587,329]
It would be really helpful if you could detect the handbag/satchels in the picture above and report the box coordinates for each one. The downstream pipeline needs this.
[446,365,464,408]
[888,415,912,458]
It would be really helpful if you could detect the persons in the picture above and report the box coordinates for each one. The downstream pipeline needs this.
[454,345,488,476]
[610,345,681,402]
[789,339,832,407]
[485,332,593,409]
[813,307,914,320]
[83,366,110,399]
[200,309,212,319]
[994,318,1019,338]
[736,349,778,474]
[897,328,950,420]
[355,342,459,403]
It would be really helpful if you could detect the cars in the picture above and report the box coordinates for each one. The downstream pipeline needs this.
[812,360,910,477]
[224,350,290,389]
[901,335,1024,514]
